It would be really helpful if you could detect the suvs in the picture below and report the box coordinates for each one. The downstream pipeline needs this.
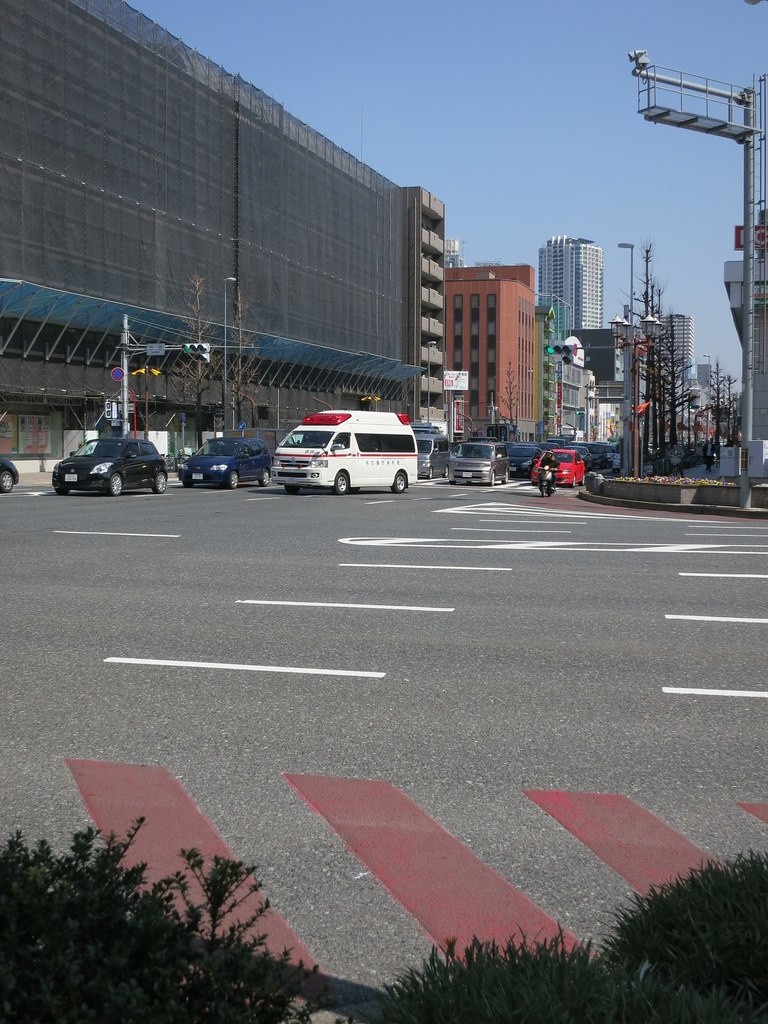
[53,438,168,496]
[178,437,272,489]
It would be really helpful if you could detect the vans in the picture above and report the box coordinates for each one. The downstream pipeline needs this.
[411,422,450,478]
[449,435,621,486]
[271,411,419,494]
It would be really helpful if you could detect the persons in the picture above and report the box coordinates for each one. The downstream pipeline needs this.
[702,434,741,474]
[665,443,686,478]
[542,449,560,486]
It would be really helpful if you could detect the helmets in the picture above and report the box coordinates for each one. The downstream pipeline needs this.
[545,449,553,453]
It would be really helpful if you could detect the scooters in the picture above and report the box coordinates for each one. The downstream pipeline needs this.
[538,465,558,498]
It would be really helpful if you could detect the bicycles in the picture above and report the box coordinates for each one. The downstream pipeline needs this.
[670,455,682,476]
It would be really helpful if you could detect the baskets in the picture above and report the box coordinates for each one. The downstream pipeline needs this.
[671,457,681,464]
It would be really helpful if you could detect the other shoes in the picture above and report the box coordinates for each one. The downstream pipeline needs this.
[551,487,554,489]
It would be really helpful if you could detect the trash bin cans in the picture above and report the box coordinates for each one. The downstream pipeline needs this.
[656,459,670,476]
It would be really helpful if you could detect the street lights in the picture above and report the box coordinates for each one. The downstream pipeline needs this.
[427,341,438,421]
[361,395,381,412]
[576,411,586,415]
[132,366,162,440]
[223,277,237,433]
[617,242,634,477]
[703,354,712,456]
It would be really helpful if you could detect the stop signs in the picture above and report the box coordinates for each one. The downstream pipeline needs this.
[697,411,704,417]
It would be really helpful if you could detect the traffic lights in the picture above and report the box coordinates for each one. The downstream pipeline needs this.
[563,355,573,365]
[544,344,575,355]
[196,354,212,362]
[689,406,699,409]
[181,342,211,354]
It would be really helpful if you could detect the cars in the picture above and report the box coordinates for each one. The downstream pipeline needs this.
[0,457,20,494]
